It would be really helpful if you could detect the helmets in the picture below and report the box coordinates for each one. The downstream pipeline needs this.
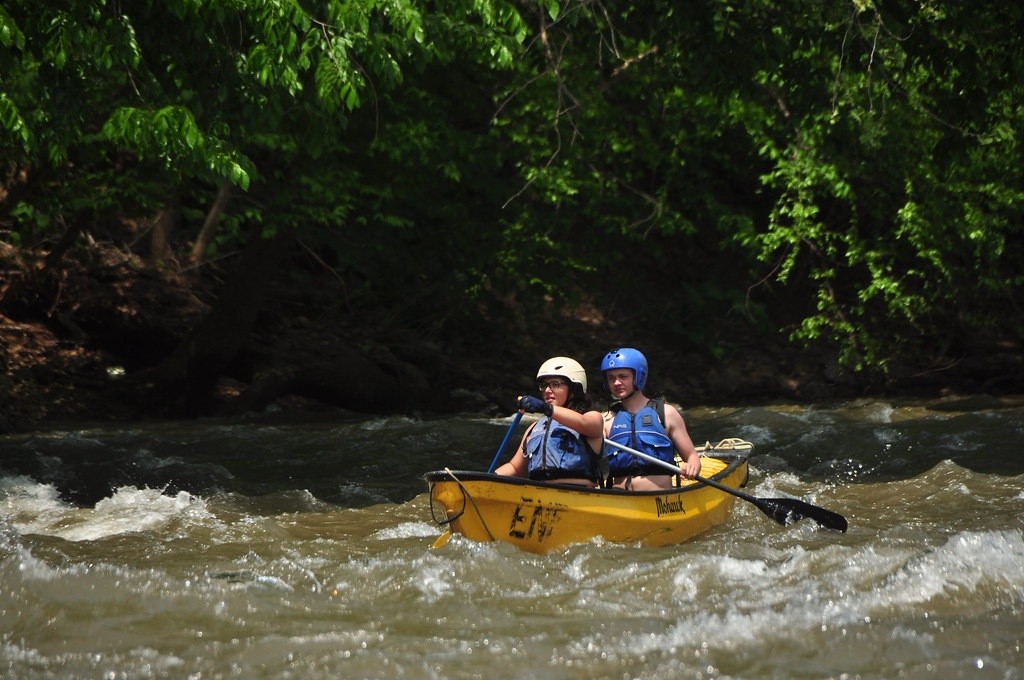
[601,348,648,394]
[536,357,587,395]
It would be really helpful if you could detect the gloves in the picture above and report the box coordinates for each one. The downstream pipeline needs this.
[517,396,553,418]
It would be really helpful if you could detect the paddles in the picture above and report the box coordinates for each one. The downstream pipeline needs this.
[432,395,526,549]
[602,437,851,535]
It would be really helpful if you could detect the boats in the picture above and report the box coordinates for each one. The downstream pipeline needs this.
[424,438,755,558]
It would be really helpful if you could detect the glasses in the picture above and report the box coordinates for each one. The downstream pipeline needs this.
[538,380,567,392]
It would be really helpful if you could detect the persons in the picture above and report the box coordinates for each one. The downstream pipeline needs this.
[494,357,604,487]
[595,348,702,490]
